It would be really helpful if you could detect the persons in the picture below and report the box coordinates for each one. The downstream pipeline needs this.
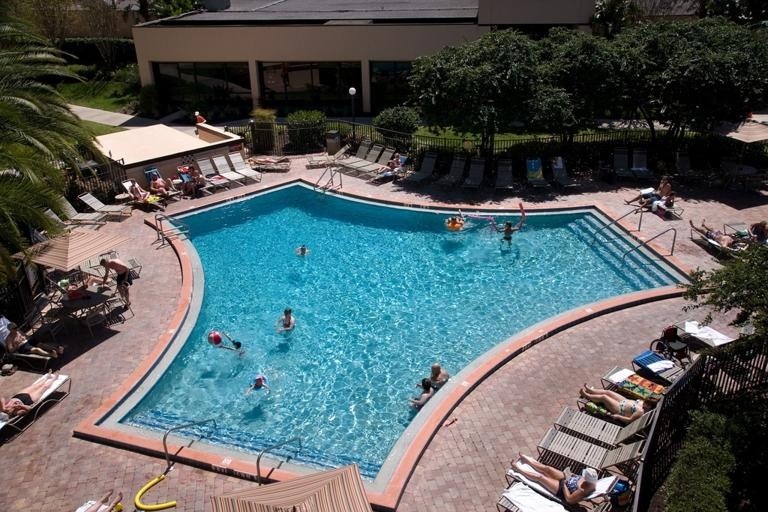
[219,331,245,357]
[447,208,464,226]
[378,152,401,175]
[244,375,269,396]
[0,369,59,418]
[5,322,64,358]
[665,191,675,220]
[623,175,673,213]
[580,382,659,422]
[247,157,289,165]
[64,275,112,292]
[99,259,133,306]
[490,217,525,241]
[409,377,435,410]
[275,307,296,333]
[84,489,124,511]
[428,363,449,390]
[150,172,177,196]
[510,451,600,505]
[689,218,741,248]
[736,220,768,245]
[194,110,207,135]
[130,178,151,202]
[297,245,309,256]
[183,165,206,199]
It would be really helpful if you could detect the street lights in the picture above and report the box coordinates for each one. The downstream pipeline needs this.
[349,86,357,149]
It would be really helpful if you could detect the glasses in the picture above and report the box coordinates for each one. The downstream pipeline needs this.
[12,327,18,329]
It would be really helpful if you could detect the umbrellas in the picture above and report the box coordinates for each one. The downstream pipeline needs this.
[8,229,135,290]
[210,461,374,512]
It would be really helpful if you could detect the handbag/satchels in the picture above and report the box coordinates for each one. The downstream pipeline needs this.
[611,480,633,507]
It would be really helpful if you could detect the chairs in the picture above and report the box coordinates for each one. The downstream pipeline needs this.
[540,425,647,467]
[640,187,685,220]
[575,318,738,425]
[0,247,144,369]
[723,222,768,244]
[44,151,292,231]
[0,372,73,440]
[690,225,750,258]
[555,403,656,446]
[506,454,620,502]
[304,137,582,195]
[633,150,657,188]
[503,478,610,512]
[612,145,633,185]
